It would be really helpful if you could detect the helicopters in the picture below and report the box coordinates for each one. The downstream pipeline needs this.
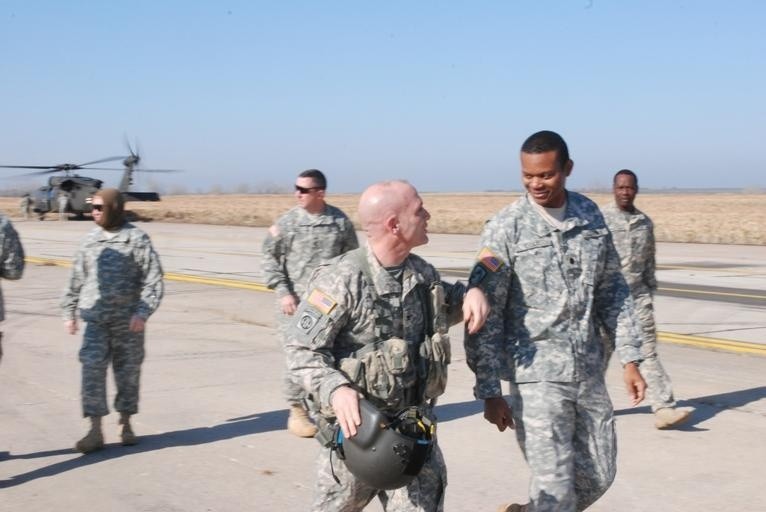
[0,130,188,221]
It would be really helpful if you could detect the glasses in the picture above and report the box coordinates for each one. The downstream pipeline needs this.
[92,205,103,211]
[296,185,324,193]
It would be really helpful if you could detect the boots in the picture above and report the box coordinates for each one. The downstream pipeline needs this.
[287,405,318,437]
[119,414,137,446]
[653,406,696,430]
[76,416,103,453]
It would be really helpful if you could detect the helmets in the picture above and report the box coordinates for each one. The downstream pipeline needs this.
[343,398,433,490]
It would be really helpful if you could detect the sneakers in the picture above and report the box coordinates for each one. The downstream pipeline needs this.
[497,503,529,512]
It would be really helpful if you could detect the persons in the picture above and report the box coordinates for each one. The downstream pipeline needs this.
[260,170,359,437]
[59,188,163,452]
[465,132,647,512]
[0,214,25,358]
[282,180,490,512]
[600,170,696,429]
